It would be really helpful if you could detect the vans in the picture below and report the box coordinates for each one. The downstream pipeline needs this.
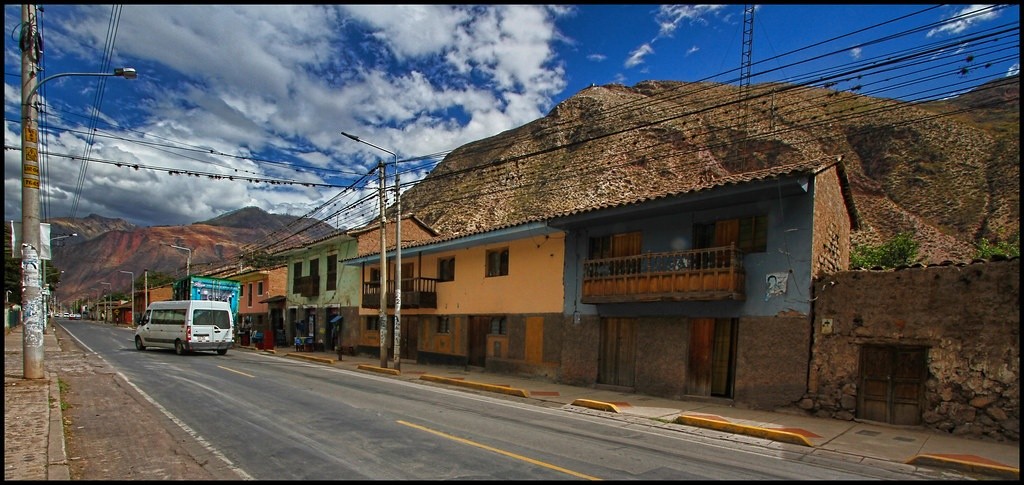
[135,300,234,356]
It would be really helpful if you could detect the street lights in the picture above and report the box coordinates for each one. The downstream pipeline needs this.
[100,282,113,322]
[19,66,139,382]
[117,270,135,329]
[341,130,401,370]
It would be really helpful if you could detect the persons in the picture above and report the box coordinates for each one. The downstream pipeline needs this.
[767,275,783,301]
[243,318,251,346]
[25,262,36,269]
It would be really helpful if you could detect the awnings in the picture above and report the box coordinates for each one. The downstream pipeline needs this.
[257,295,288,306]
[328,315,344,324]
